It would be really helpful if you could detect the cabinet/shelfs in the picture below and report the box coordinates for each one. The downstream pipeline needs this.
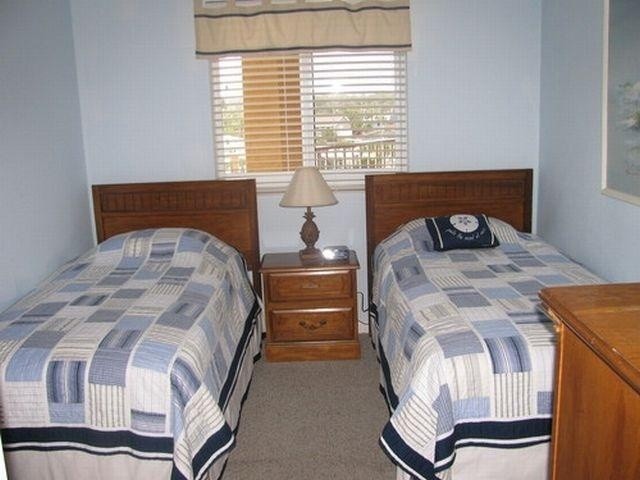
[535,281,640,480]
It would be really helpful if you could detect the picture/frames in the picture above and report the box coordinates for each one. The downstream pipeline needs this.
[600,0,640,206]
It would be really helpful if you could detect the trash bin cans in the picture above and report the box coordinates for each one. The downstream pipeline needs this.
[362,159,375,168]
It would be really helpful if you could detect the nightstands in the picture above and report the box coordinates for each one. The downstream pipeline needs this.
[256,249,362,361]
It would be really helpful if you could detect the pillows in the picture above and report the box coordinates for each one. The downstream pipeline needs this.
[425,212,500,251]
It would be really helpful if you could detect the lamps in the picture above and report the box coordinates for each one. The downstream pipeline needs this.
[278,166,339,260]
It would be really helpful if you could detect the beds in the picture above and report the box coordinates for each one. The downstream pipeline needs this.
[0,178,268,479]
[365,168,612,480]
